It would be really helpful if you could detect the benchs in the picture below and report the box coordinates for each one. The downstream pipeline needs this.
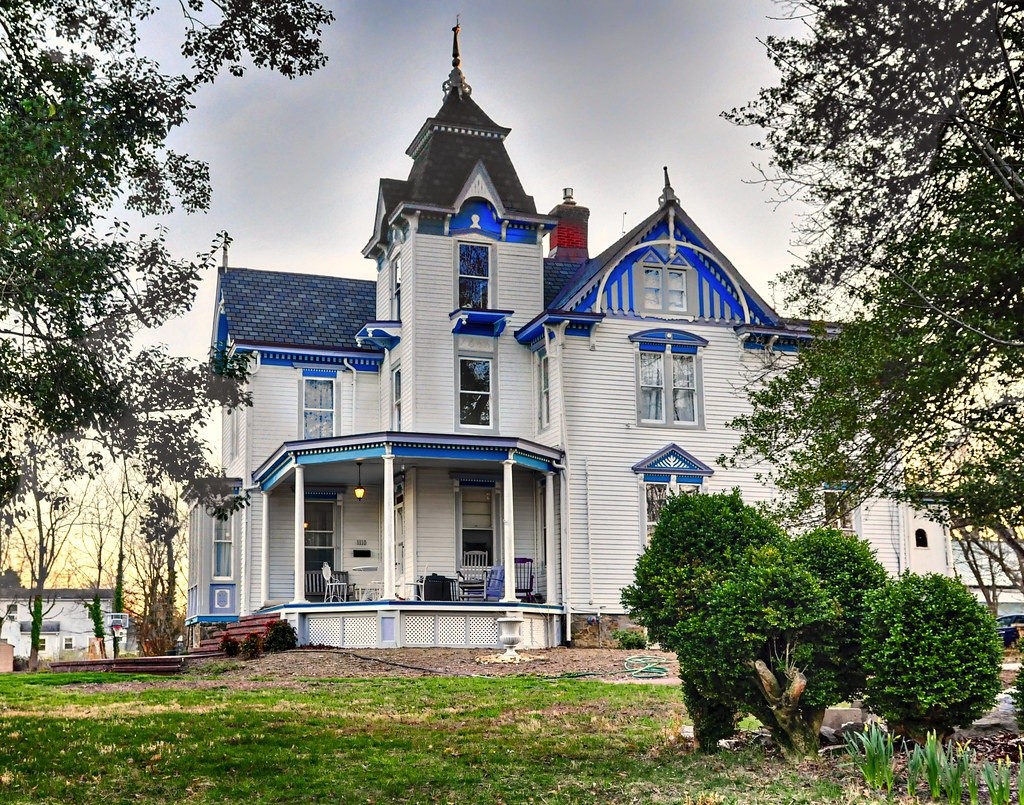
[305,570,355,602]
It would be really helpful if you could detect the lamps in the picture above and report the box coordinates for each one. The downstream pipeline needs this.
[355,462,364,499]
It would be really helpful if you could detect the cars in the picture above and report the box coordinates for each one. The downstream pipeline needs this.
[995,614,1024,647]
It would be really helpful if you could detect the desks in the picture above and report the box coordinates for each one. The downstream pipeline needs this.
[352,566,383,601]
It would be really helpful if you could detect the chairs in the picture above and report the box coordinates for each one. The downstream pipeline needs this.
[404,564,428,600]
[468,567,504,601]
[455,551,487,601]
[322,562,347,603]
[513,558,534,603]
